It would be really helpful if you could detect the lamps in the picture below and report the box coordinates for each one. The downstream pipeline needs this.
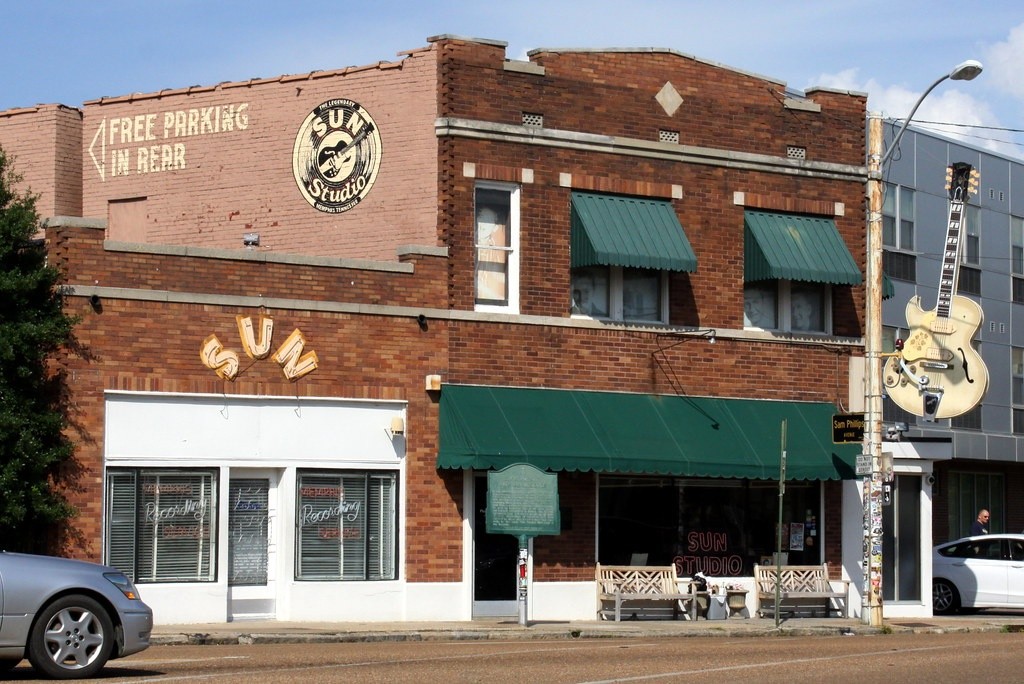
[895,421,908,437]
[652,330,716,357]
[243,234,261,248]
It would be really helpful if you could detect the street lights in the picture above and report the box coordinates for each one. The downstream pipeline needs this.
[860,60,987,625]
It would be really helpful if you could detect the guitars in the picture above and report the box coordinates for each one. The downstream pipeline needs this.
[883,160,990,422]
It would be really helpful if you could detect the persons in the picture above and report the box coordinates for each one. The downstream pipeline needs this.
[790,289,820,333]
[744,287,775,329]
[969,509,1001,558]
[570,268,607,317]
[623,277,658,317]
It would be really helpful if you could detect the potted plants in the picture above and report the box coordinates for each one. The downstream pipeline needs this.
[730,583,751,620]
[689,577,709,624]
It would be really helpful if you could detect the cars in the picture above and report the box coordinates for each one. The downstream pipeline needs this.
[0,550,153,681]
[932,534,1024,617]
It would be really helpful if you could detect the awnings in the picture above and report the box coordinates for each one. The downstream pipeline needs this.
[435,383,872,481]
[744,211,863,286]
[882,271,895,300]
[571,191,698,272]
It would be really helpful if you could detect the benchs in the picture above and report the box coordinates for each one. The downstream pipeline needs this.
[755,562,854,620]
[597,562,702,622]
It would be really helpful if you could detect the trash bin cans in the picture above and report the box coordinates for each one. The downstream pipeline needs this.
[706,594,726,619]
